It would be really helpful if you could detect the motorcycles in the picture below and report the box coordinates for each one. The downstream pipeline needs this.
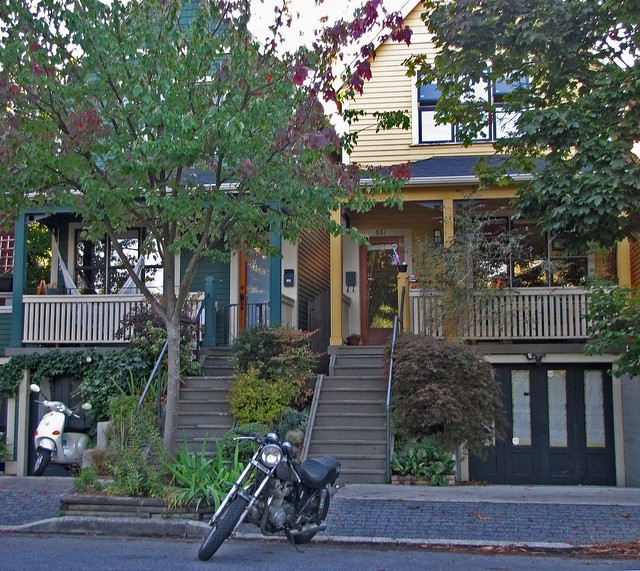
[198,432,341,562]
[29,383,94,477]
